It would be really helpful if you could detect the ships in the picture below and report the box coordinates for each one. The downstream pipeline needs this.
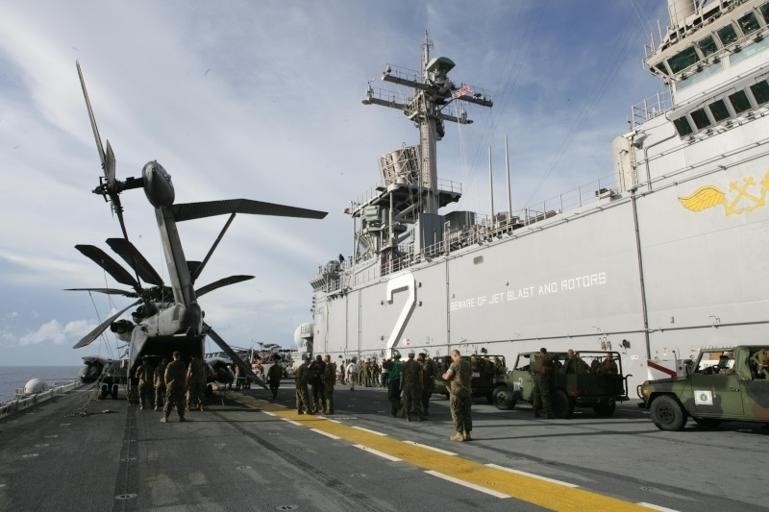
[0,2,768,509]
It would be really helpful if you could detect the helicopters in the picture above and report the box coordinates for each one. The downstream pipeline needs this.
[62,55,329,403]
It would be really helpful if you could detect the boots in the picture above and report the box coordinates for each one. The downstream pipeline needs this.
[449,431,473,442]
[178,415,188,423]
[159,414,170,424]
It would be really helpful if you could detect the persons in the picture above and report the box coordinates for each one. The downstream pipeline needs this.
[472,348,502,370]
[267,358,282,401]
[531,347,554,419]
[440,350,473,442]
[134,350,206,422]
[294,352,439,423]
[558,349,619,376]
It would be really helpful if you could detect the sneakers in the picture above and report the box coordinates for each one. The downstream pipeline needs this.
[389,407,433,423]
[138,403,207,414]
[296,407,335,416]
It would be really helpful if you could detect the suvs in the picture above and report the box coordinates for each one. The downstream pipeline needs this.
[427,353,507,403]
[491,351,631,419]
[634,344,769,431]
